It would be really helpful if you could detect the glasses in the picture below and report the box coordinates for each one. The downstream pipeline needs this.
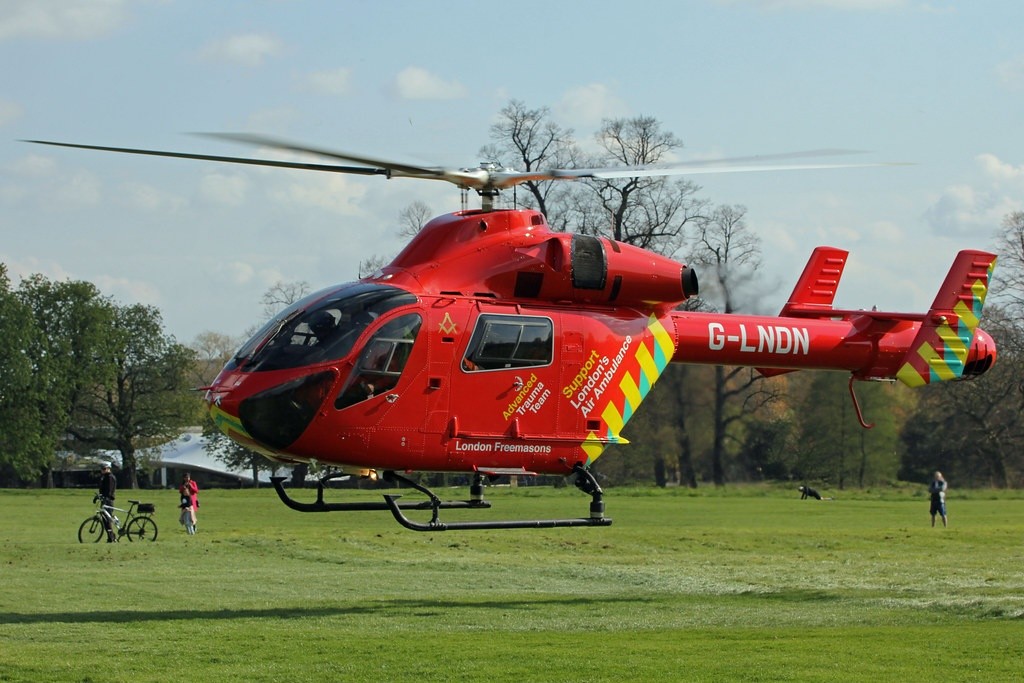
[102,468,106,470]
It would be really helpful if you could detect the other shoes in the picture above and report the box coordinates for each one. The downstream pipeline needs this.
[107,538,115,543]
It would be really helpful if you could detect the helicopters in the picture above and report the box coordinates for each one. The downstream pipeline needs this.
[20,132,997,530]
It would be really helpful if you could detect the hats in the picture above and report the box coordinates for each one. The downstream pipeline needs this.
[97,461,112,468]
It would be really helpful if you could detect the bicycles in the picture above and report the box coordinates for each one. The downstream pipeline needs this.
[78,493,158,543]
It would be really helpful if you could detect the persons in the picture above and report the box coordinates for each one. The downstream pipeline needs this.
[798,485,833,500]
[929,471,947,528]
[278,303,399,414]
[99,461,116,543]
[178,473,200,535]
